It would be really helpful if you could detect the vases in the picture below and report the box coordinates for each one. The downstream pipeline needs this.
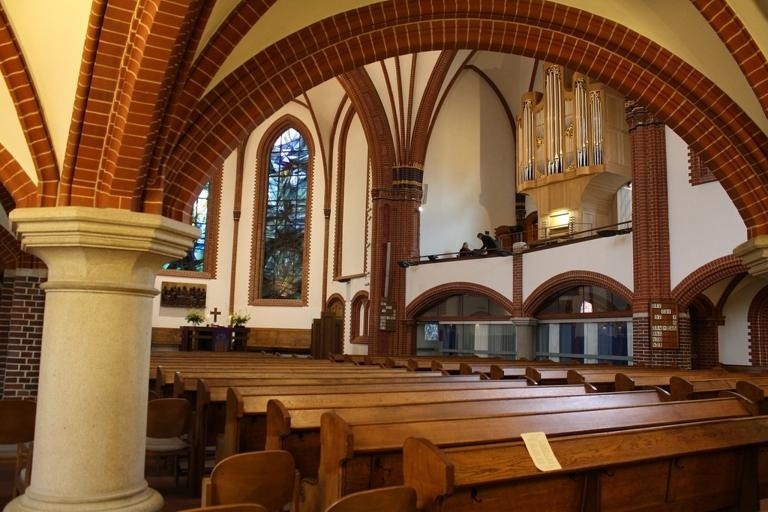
[192,321,196,326]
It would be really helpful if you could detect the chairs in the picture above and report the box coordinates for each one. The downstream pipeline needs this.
[0,400,36,498]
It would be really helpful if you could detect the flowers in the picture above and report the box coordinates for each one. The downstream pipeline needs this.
[184,311,208,325]
[229,313,251,323]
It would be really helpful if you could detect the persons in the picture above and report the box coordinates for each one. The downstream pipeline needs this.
[460,243,470,256]
[477,233,496,253]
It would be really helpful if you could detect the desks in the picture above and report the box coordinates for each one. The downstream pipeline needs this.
[180,326,251,352]
